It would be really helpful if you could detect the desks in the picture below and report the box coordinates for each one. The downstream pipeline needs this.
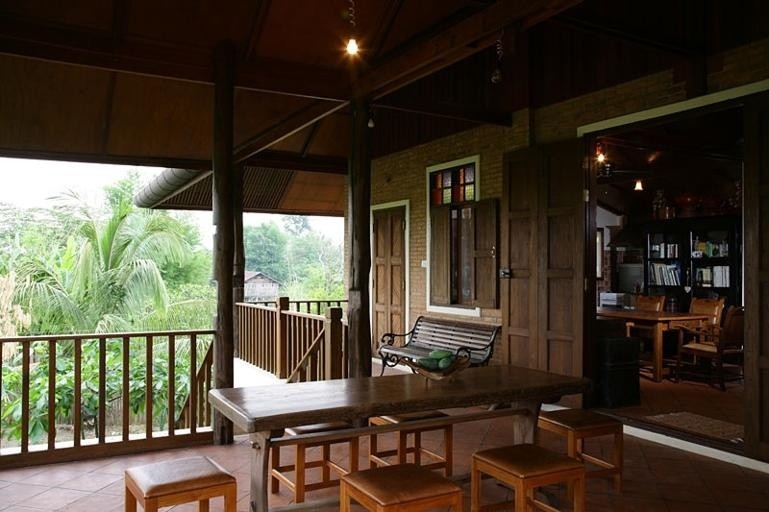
[596,307,707,382]
[208,364,589,511]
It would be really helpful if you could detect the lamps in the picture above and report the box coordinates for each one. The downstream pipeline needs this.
[633,179,644,191]
[345,1,359,56]
[489,29,504,82]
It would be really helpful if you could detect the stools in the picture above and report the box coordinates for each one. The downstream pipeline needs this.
[538,406,623,502]
[270,423,358,504]
[125,455,237,511]
[369,412,453,478]
[471,444,588,511]
[340,463,463,511]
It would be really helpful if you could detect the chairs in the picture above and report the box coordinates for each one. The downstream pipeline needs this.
[689,296,724,341]
[643,223,739,309]
[674,305,744,391]
[636,294,666,312]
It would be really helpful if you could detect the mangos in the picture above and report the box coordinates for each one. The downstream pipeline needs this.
[420,350,452,369]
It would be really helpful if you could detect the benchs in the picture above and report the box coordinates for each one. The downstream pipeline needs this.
[379,316,501,378]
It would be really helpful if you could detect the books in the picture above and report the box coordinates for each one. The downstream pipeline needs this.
[599,292,625,311]
[649,242,730,288]
